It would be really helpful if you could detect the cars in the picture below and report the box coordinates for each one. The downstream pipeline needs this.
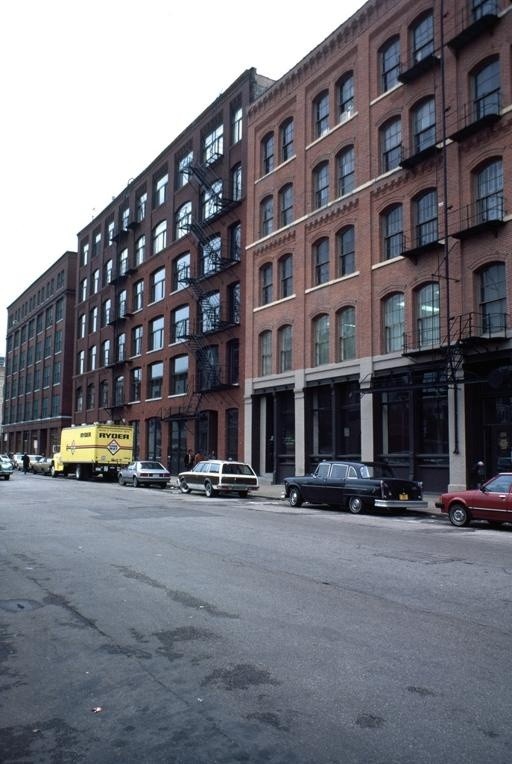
[433,471,512,528]
[118,460,170,488]
[0,451,55,480]
[281,461,429,516]
[175,459,260,496]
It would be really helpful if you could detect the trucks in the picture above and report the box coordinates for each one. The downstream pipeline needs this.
[49,420,137,482]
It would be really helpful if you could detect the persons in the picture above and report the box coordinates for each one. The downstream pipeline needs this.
[184,448,195,471]
[22,451,30,475]
[207,449,216,460]
[193,450,204,464]
